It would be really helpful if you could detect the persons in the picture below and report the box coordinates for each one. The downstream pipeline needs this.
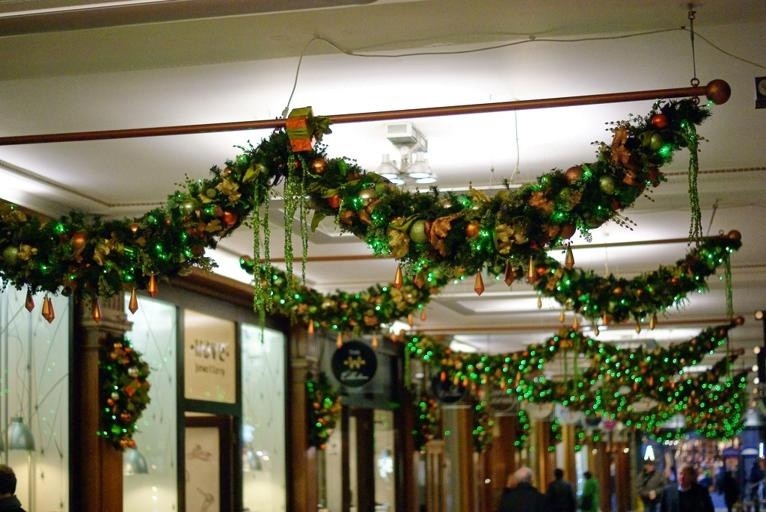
[0,464,25,512]
[493,451,766,511]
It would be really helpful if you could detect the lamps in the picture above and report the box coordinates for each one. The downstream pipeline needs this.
[371,123,438,189]
